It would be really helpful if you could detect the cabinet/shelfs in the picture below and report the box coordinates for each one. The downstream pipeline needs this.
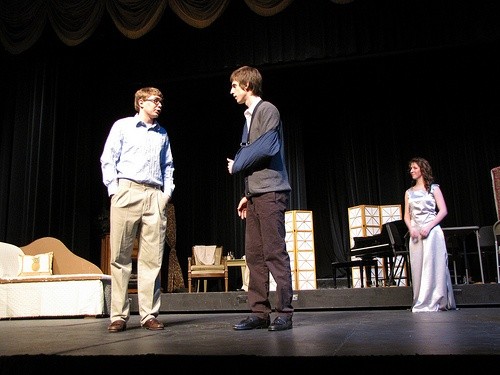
[101,233,140,294]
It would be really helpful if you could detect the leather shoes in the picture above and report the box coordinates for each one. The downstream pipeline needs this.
[107,320,128,333]
[232,315,271,331]
[267,316,294,331]
[142,318,164,331]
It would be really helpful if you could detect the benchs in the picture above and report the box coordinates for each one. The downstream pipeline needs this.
[331,260,379,289]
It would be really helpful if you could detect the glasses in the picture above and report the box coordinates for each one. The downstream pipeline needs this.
[143,99,162,105]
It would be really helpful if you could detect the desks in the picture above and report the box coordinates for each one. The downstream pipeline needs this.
[225,258,246,286]
[441,226,485,283]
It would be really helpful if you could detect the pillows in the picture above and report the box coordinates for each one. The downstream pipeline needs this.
[17,251,54,277]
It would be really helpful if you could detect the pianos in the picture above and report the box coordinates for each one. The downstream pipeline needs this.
[348,221,412,286]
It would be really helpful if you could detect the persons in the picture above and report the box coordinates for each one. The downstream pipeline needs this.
[227,66,293,331]
[99,88,176,333]
[403,158,457,313]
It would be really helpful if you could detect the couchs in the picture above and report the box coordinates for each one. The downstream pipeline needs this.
[0,237,112,319]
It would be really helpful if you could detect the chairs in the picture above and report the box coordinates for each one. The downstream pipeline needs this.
[493,220,500,283]
[188,245,229,293]
